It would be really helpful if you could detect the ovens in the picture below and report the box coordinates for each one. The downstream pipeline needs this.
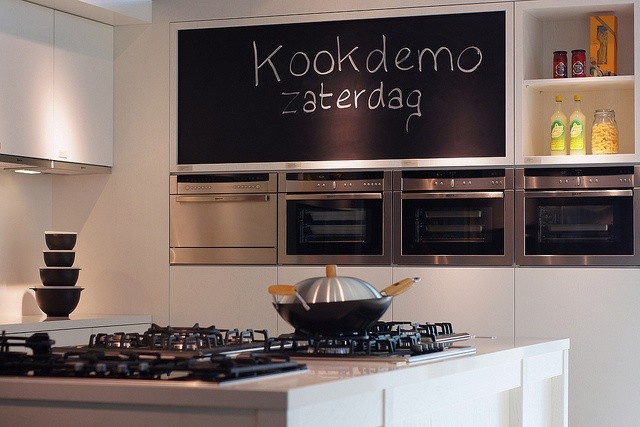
[169,170,277,264]
[515,163,639,269]
[278,168,393,264]
[393,166,515,266]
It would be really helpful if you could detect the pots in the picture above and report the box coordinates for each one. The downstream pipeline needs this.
[269,265,421,337]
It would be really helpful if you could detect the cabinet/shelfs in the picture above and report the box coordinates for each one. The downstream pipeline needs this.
[514,1,639,164]
[1,1,115,167]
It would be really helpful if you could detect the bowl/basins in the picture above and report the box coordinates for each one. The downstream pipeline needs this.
[43,250,76,267]
[39,268,81,285]
[30,286,85,321]
[44,232,77,250]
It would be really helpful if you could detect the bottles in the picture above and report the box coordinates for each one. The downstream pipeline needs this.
[551,95,567,156]
[570,96,587,156]
[592,109,618,155]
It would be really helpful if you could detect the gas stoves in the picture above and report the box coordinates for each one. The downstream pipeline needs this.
[261,318,496,357]
[0,327,309,387]
[86,320,268,355]
